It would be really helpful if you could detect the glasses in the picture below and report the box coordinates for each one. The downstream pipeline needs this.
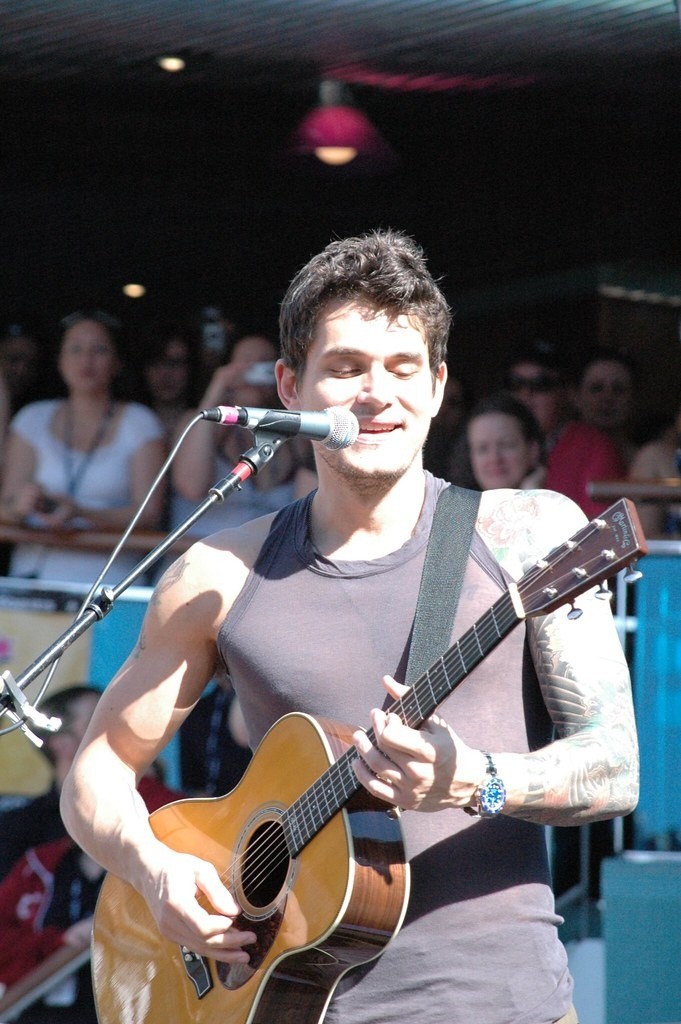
[504,373,561,392]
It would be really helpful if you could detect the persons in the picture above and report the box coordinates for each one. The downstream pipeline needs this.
[0,306,681,1024]
[57,227,642,1024]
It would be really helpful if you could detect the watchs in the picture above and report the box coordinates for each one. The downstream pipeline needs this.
[462,749,506,818]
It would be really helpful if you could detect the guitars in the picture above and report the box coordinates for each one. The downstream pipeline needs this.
[89,492,653,1024]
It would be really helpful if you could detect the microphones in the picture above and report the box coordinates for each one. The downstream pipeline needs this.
[200,404,360,450]
